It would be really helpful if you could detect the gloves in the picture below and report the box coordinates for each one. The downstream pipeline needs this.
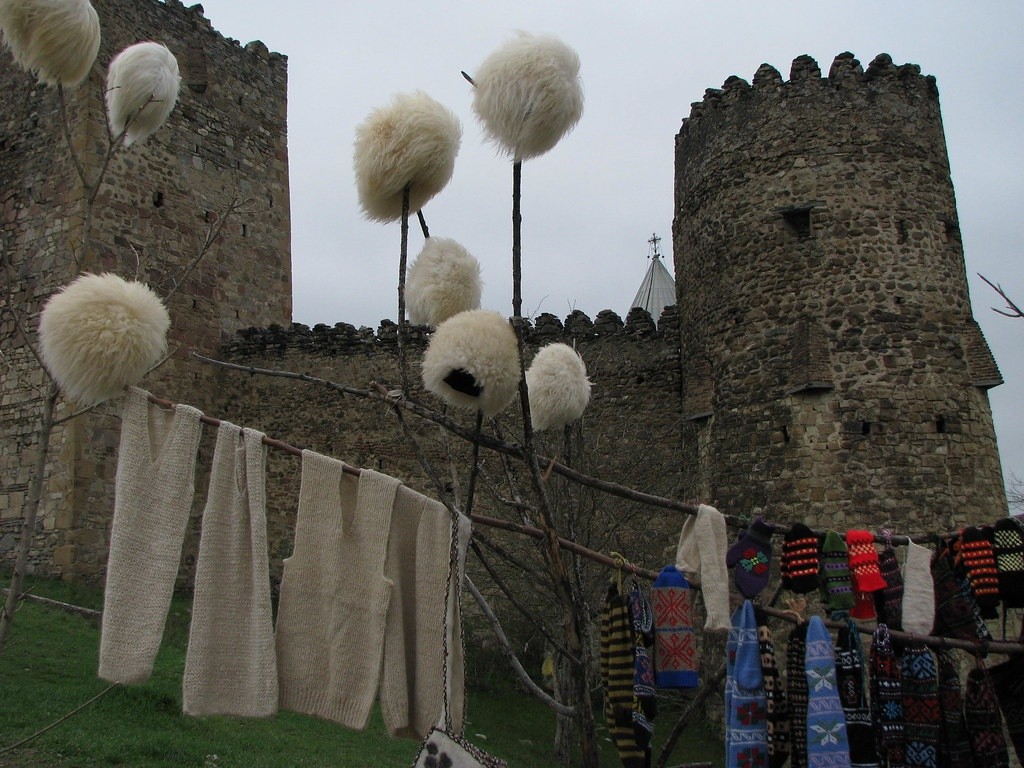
[725,517,1024,639]
